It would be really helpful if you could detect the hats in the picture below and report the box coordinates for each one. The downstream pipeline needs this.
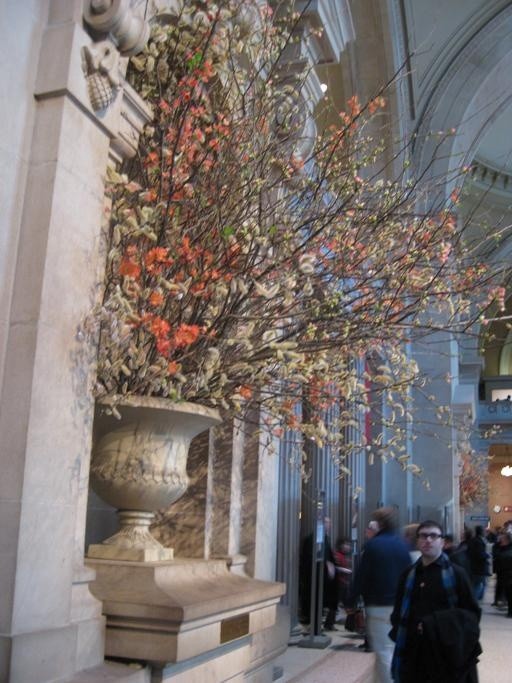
[368,521,380,532]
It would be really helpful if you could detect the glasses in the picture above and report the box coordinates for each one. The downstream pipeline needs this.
[420,533,441,539]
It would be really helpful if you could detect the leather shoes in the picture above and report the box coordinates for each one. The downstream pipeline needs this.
[359,642,373,652]
[325,623,338,631]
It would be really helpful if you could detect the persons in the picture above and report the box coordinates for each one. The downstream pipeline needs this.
[358,505,415,683]
[297,505,512,654]
[386,519,485,683]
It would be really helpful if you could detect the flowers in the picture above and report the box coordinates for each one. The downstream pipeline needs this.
[74,0,511,487]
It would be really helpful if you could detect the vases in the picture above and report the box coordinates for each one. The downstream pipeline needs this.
[91,393,221,569]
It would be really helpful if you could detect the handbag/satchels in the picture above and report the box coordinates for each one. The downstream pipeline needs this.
[345,610,364,634]
